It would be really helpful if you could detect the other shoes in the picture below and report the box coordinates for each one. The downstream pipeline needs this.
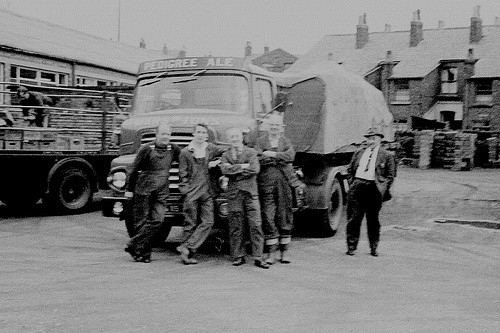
[126,240,151,263]
[176,243,197,265]
[255,259,270,269]
[233,256,246,266]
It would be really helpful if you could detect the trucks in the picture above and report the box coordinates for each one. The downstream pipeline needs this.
[0,76,135,217]
[100,54,398,239]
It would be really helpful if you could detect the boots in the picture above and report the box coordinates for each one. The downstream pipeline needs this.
[265,237,279,264]
[281,238,291,262]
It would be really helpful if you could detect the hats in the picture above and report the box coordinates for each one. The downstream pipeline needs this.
[364,126,384,139]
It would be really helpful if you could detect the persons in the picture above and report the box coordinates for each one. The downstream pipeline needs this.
[15,87,53,127]
[176,124,223,264]
[124,122,181,262]
[255,115,305,265]
[218,128,270,268]
[345,127,394,257]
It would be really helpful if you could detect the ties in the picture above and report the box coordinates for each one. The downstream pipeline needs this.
[365,147,373,172]
